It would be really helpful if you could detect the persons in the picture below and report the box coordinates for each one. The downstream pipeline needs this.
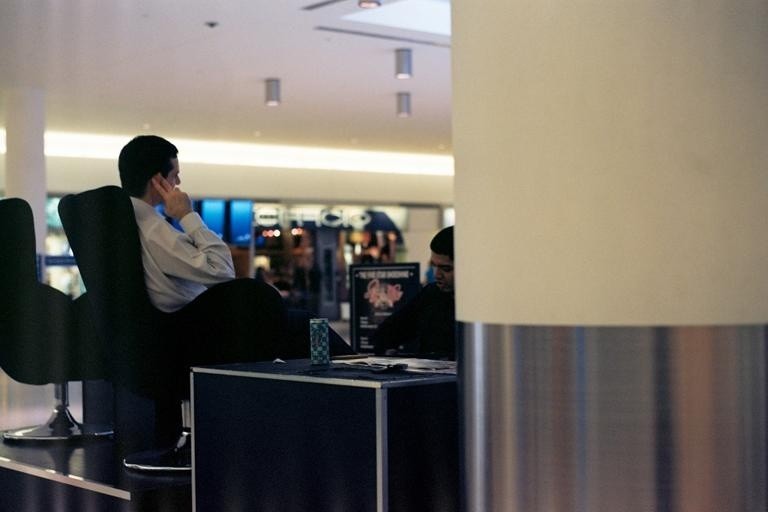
[362,228,398,264]
[371,224,454,363]
[117,133,357,357]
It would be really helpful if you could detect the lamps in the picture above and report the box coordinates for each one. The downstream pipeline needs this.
[395,49,411,82]
[264,77,280,107]
[397,91,411,117]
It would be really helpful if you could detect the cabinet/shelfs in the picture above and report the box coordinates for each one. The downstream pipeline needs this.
[188,355,459,512]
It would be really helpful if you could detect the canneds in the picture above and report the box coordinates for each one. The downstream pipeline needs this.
[309,318,330,366]
